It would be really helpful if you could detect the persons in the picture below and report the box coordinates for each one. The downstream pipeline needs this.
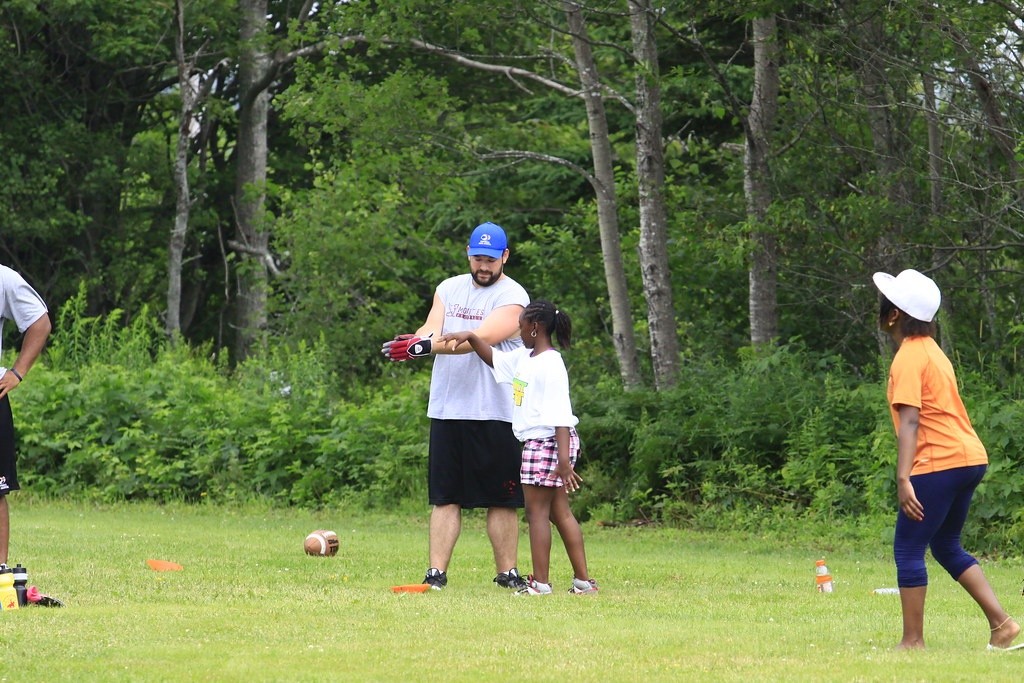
[0,260,53,578]
[381,221,531,595]
[870,267,1023,656]
[438,301,600,598]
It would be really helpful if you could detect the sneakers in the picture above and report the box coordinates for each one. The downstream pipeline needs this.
[422,568,448,590]
[514,574,553,595]
[568,579,599,594]
[493,568,529,589]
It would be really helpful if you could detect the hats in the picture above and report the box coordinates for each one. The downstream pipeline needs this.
[467,222,507,258]
[873,269,941,323]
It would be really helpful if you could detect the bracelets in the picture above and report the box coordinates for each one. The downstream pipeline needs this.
[10,368,24,383]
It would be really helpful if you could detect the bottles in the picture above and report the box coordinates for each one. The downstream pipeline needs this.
[814,559,835,596]
[0,564,28,609]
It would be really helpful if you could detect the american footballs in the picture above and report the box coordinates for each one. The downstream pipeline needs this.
[305,529,339,556]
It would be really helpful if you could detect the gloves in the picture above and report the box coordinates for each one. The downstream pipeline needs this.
[381,333,434,362]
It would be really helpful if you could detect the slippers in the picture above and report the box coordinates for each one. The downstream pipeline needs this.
[988,644,1024,650]
